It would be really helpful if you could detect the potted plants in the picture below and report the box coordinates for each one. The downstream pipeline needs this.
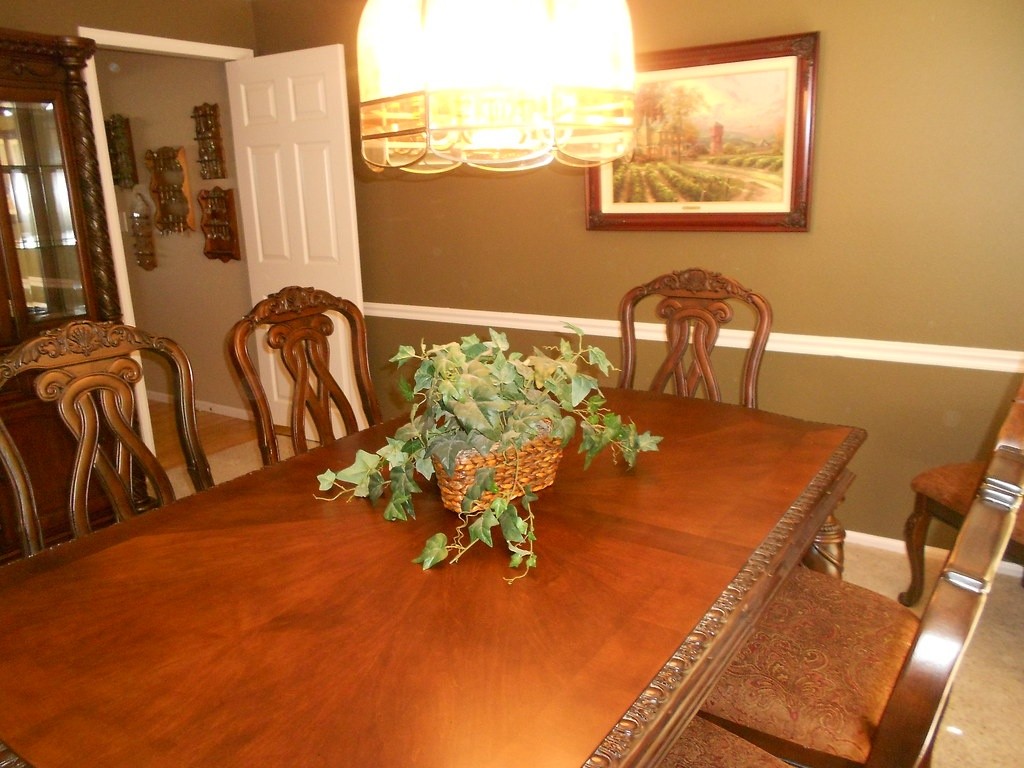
[318,320,665,585]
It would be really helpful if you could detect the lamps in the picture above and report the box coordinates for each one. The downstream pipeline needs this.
[351,1,633,178]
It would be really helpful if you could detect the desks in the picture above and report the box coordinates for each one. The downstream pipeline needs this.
[0,387,865,767]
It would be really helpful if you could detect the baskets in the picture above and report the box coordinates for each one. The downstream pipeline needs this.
[432,416,563,512]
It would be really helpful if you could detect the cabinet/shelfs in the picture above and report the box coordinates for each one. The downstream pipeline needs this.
[0,27,160,560]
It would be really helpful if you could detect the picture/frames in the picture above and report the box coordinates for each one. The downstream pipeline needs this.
[583,29,821,233]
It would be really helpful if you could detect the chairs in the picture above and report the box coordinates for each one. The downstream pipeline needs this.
[616,268,773,409]
[898,385,1024,610]
[229,286,385,467]
[656,392,1023,767]
[1,319,219,563]
